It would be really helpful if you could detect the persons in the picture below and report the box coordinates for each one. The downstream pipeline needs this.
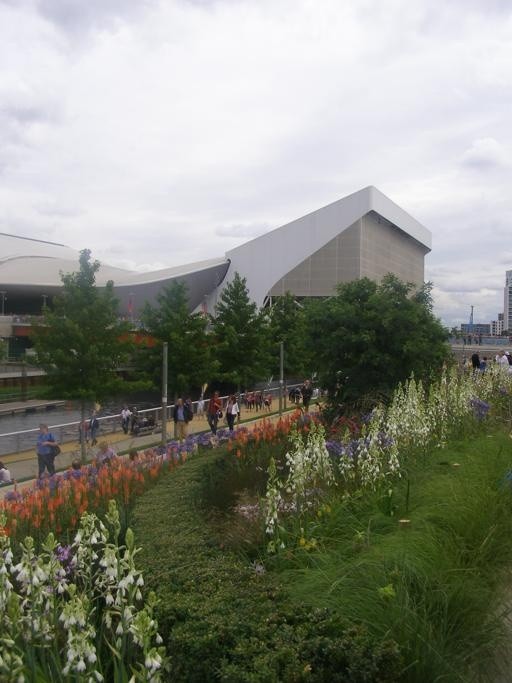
[35,421,59,478]
[0,460,13,484]
[71,377,326,471]
[461,332,512,376]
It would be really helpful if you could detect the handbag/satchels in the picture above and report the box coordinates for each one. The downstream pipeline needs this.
[48,432,61,459]
[216,408,224,419]
